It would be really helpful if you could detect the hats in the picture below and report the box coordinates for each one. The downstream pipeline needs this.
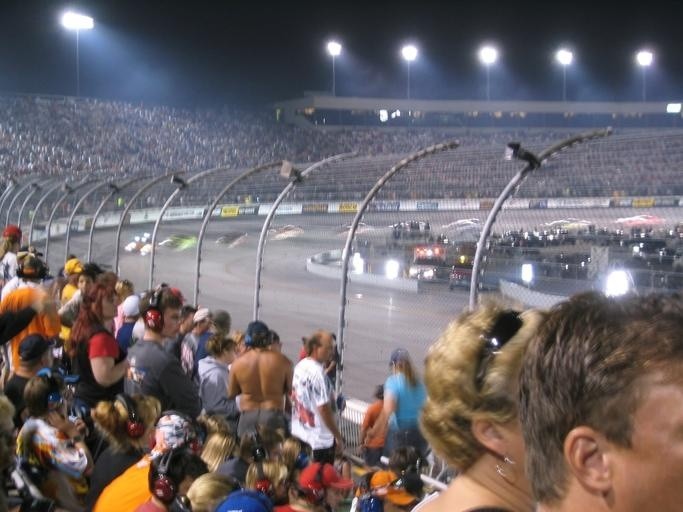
[150,415,196,456]
[121,295,140,317]
[19,334,55,360]
[299,462,354,489]
[23,259,42,275]
[4,225,21,239]
[83,265,103,273]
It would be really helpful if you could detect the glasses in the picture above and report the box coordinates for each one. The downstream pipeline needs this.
[475,309,522,393]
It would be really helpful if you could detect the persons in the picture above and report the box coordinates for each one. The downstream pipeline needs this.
[2,220,352,512]
[391,216,682,290]
[354,291,683,511]
[2,89,682,224]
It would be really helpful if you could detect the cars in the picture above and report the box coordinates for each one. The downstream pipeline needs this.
[348,235,682,293]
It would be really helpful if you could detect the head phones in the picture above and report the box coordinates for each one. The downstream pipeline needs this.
[15,264,48,281]
[117,393,144,439]
[248,426,385,511]
[144,285,163,331]
[46,373,60,411]
[150,452,173,502]
[245,321,274,346]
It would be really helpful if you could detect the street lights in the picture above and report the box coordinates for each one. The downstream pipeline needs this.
[327,39,343,97]
[554,49,572,100]
[401,43,420,97]
[60,10,95,94]
[479,46,499,99]
[635,50,655,102]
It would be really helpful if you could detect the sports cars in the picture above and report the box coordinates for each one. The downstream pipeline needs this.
[614,214,666,229]
[269,225,304,240]
[125,233,158,256]
[161,233,196,252]
[338,222,373,236]
[442,217,481,233]
[536,216,595,234]
[214,232,249,248]
[388,220,431,233]
